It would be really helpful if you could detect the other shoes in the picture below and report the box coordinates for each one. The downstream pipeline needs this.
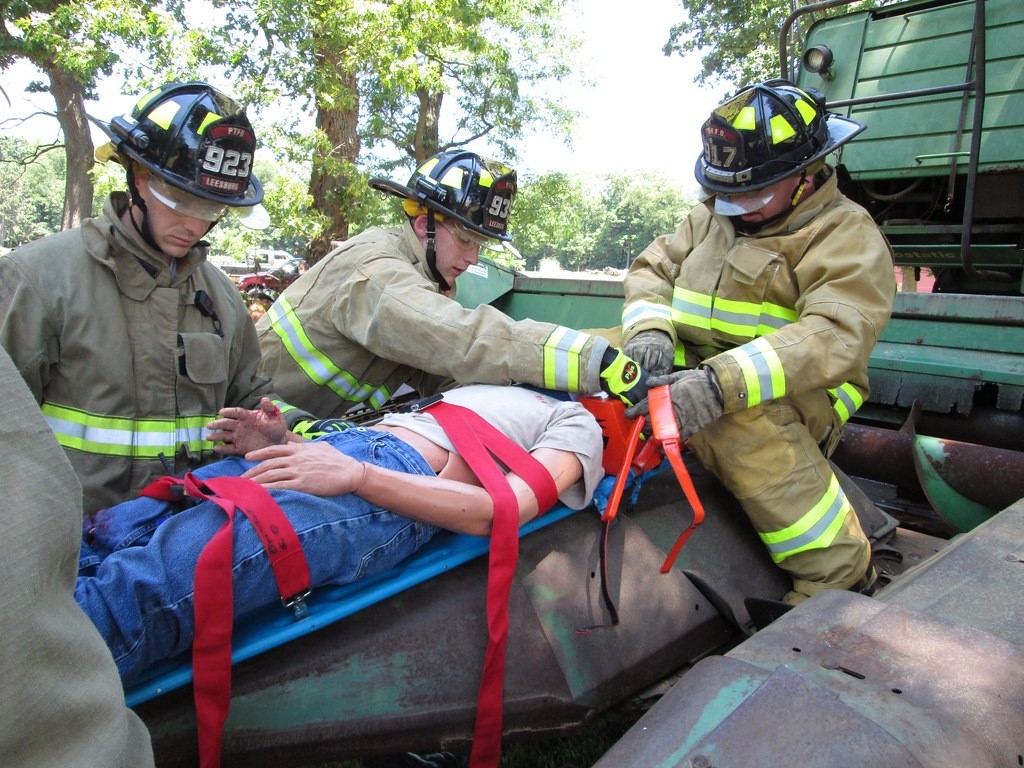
[750,561,877,635]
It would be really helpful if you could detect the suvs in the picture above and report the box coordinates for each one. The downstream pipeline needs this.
[233,256,303,292]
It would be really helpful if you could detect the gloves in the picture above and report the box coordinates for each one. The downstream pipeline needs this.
[292,418,360,440]
[623,328,674,375]
[624,366,723,456]
[600,347,654,441]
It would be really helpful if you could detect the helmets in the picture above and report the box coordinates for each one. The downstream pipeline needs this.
[367,150,517,241]
[694,80,868,192]
[84,81,264,207]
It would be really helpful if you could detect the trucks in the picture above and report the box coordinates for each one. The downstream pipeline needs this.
[223,251,295,271]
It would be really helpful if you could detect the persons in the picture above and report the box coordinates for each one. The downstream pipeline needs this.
[621,79,898,607]
[0,342,154,768]
[74,381,604,687]
[0,81,358,513]
[257,150,653,421]
